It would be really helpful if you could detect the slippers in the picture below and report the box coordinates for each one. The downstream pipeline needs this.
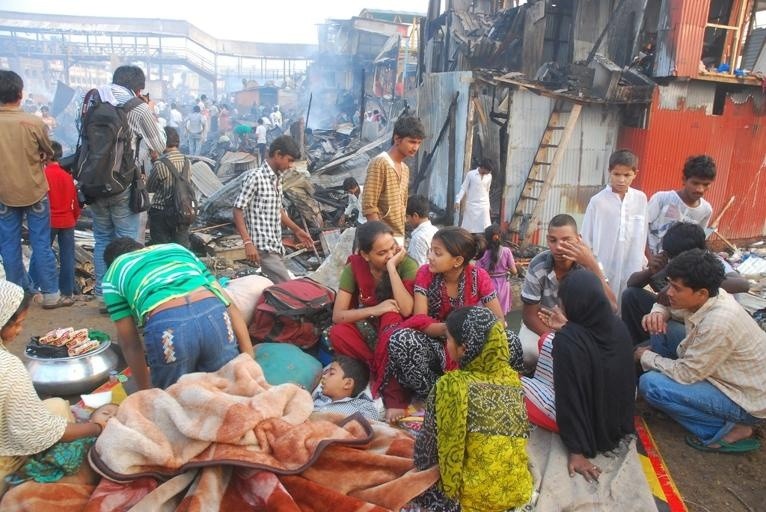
[43,289,74,309]
[686,424,760,455]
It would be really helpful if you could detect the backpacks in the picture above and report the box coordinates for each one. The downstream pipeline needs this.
[154,158,198,226]
[73,88,144,196]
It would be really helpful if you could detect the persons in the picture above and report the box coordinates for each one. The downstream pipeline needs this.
[518,214,620,368]
[580,149,652,315]
[646,154,719,271]
[516,269,639,484]
[3,56,532,510]
[620,221,750,401]
[636,248,766,452]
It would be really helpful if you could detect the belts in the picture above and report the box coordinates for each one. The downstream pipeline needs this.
[149,290,217,317]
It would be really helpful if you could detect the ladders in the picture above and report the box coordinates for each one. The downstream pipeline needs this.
[502,96,582,258]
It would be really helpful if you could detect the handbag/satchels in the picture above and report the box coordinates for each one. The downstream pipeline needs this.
[249,277,336,350]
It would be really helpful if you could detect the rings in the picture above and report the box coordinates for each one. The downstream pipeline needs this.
[591,465,597,471]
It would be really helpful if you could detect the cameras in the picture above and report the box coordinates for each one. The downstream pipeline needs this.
[139,93,149,103]
